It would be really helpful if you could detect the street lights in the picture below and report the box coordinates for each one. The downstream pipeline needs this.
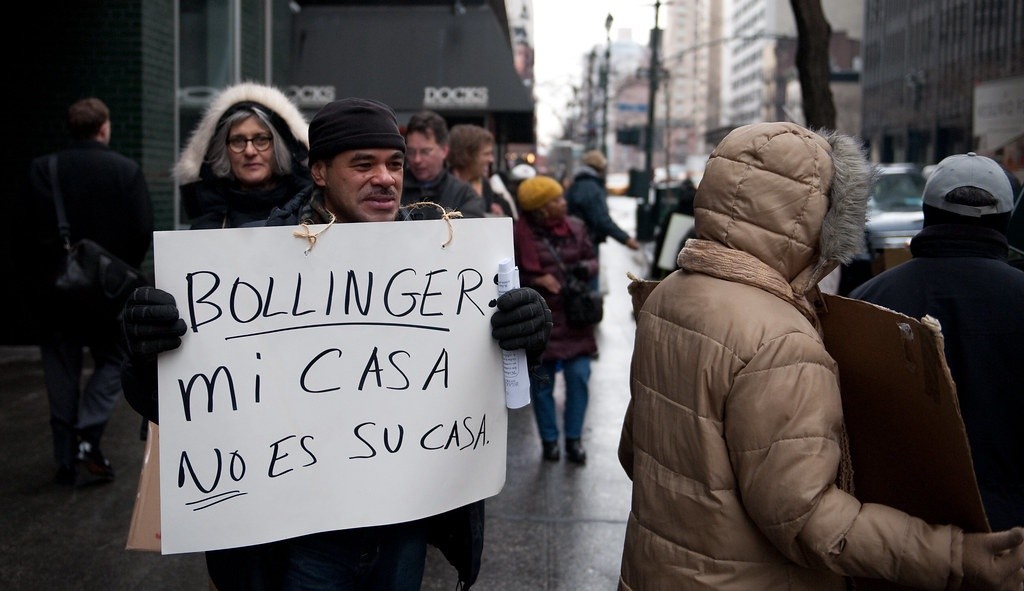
[571,0,666,201]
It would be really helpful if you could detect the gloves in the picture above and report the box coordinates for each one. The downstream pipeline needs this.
[490,287,553,366]
[120,286,186,377]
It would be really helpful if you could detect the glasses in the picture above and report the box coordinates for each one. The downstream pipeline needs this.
[226,136,272,153]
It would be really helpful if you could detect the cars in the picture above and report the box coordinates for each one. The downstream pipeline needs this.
[863,163,928,248]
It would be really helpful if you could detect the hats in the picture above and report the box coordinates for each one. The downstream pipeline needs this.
[512,164,535,180]
[921,152,1013,218]
[308,97,406,169]
[583,150,606,169]
[518,177,563,209]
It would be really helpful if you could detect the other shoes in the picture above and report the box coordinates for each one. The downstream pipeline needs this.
[543,440,560,460]
[566,438,585,461]
[74,437,114,482]
[50,472,85,490]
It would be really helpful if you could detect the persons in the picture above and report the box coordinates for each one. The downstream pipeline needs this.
[836,150,1024,533]
[121,100,552,591]
[176,82,313,229]
[618,122,1024,591]
[514,175,604,464]
[24,97,155,492]
[401,112,697,324]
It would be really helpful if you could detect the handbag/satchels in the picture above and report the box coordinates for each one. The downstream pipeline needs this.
[56,239,141,302]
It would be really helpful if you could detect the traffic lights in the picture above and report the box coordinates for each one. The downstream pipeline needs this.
[616,126,642,146]
